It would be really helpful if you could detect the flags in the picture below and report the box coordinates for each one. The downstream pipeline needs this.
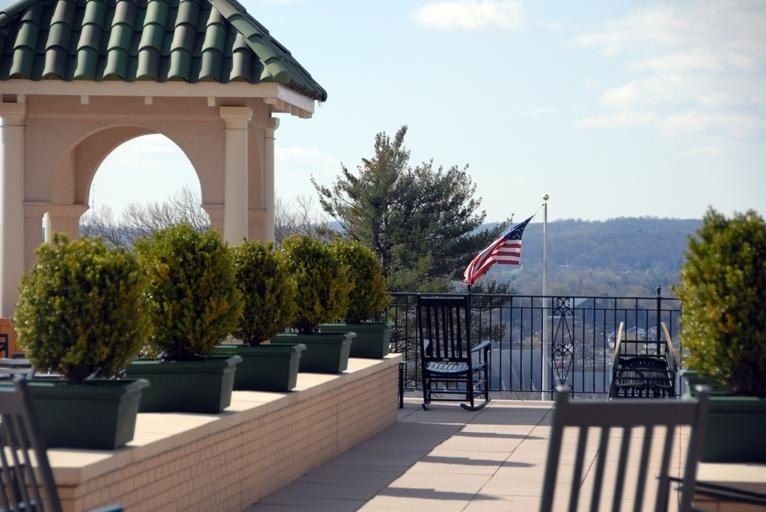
[463,215,533,286]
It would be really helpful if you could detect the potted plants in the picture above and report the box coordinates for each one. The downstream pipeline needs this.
[128,218,243,414]
[270,232,357,375]
[318,238,391,360]
[1,230,150,451]
[669,205,766,464]
[213,237,307,393]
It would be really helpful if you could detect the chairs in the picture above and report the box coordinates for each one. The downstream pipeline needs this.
[0,371,124,512]
[416,292,492,411]
[607,320,679,398]
[539,384,711,512]
